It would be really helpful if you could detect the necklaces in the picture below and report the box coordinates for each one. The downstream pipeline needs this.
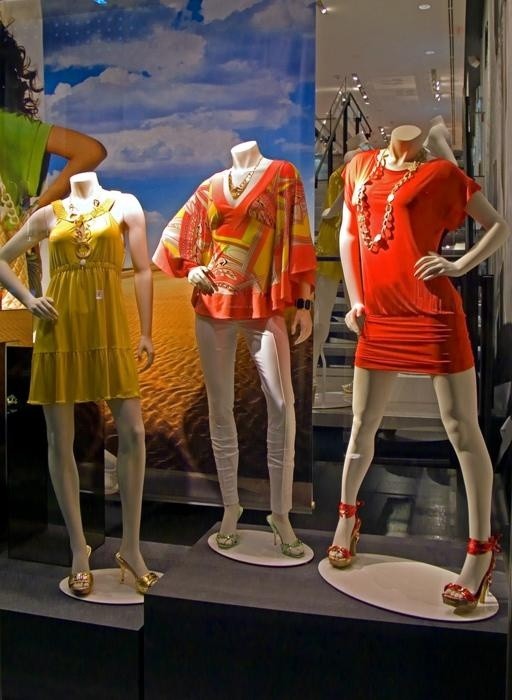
[355,145,429,251]
[67,190,103,267]
[227,156,265,199]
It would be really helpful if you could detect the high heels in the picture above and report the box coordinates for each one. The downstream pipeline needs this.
[216,506,244,549]
[69,546,94,597]
[266,514,305,557]
[326,500,364,567]
[115,552,158,594]
[442,536,501,610]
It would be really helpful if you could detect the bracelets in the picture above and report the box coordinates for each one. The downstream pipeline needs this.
[295,298,312,312]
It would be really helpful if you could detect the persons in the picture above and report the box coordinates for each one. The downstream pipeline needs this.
[313,131,374,394]
[0,18,108,221]
[328,126,512,611]
[152,141,319,559]
[0,172,159,595]
[423,114,460,166]
